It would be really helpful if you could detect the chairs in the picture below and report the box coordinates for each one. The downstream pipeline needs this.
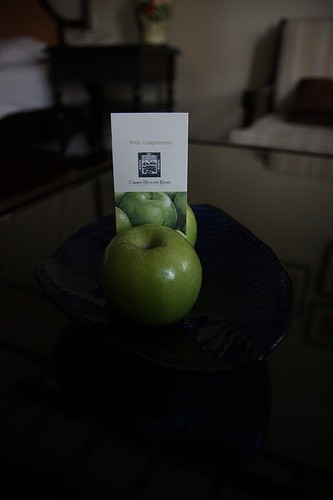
[228,16,333,154]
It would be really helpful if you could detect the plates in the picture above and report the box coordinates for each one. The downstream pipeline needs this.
[36,203,295,374]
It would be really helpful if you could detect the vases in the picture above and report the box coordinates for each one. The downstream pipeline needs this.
[144,21,169,46]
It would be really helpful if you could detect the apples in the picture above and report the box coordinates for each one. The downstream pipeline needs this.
[114,191,187,235]
[100,203,203,327]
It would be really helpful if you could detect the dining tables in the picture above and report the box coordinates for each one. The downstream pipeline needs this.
[42,45,182,163]
[1,139,332,500]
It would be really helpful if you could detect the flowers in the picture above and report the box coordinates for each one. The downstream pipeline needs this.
[138,0,175,29]
